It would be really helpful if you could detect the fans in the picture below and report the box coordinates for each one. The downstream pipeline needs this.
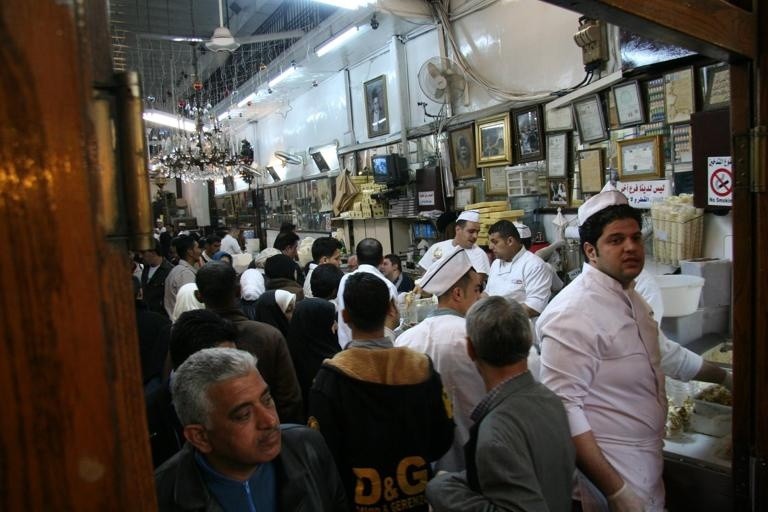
[417,54,470,107]
[137,0,305,57]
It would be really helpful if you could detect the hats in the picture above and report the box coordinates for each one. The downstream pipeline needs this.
[457,209,481,224]
[513,221,532,238]
[574,181,629,226]
[413,244,473,298]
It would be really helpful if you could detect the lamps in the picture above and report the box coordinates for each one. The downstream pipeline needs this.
[313,13,384,59]
[141,60,304,185]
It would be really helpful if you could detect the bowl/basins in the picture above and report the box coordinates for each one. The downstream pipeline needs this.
[650,274,706,318]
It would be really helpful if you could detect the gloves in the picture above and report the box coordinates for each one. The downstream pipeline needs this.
[723,370,734,391]
[607,482,647,511]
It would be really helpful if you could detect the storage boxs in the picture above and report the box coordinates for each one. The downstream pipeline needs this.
[161,190,211,237]
[659,307,703,347]
[678,255,732,307]
[696,305,730,336]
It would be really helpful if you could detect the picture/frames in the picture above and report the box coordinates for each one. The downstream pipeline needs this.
[362,73,390,139]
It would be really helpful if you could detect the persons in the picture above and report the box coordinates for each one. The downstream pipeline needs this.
[368,87,386,132]
[457,135,471,170]
[495,138,504,155]
[536,182,733,511]
[129,210,453,512]
[392,211,574,512]
[550,180,566,203]
[379,162,386,175]
[484,128,499,157]
[634,269,663,329]
[519,123,539,153]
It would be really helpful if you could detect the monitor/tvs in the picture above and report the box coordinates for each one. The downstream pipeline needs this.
[371,153,408,185]
